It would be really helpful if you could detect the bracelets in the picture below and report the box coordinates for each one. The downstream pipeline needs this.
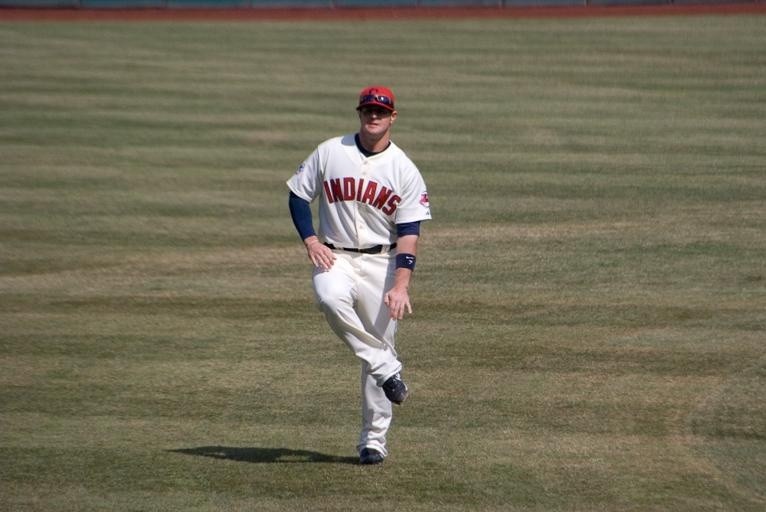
[396,253,416,272]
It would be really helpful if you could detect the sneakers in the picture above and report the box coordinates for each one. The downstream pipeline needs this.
[382,370,408,406]
[358,445,384,464]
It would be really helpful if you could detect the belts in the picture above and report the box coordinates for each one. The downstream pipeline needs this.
[324,242,397,254]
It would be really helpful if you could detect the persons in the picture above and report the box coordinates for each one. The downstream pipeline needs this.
[285,85,433,465]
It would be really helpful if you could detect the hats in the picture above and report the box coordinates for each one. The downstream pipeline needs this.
[355,86,396,112]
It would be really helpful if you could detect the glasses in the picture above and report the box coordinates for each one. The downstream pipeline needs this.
[359,94,393,108]
